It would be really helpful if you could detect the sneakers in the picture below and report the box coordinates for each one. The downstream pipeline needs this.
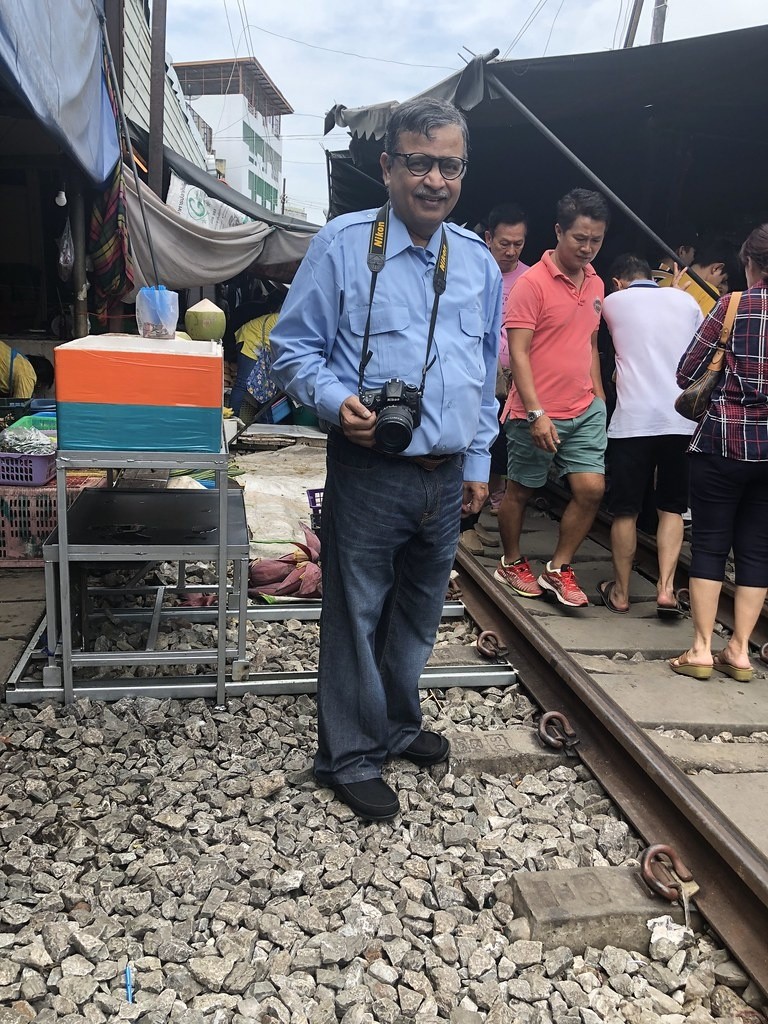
[537,560,589,608]
[493,554,543,597]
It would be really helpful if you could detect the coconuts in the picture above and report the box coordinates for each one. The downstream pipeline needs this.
[184,297,226,342]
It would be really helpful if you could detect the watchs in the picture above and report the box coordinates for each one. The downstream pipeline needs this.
[526,408,545,422]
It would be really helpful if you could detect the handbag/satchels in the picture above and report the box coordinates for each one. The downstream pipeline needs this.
[675,290,742,420]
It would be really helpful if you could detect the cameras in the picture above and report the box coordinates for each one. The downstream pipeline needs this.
[359,378,421,453]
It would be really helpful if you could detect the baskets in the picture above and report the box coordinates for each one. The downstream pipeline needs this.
[0,398,57,486]
[0,467,124,568]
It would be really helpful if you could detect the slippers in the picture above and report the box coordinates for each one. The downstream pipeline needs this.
[713,647,754,681]
[656,600,684,615]
[596,579,630,613]
[669,649,713,679]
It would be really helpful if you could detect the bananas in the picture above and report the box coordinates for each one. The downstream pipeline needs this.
[223,407,235,419]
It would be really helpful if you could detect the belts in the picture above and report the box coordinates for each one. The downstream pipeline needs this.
[414,455,450,471]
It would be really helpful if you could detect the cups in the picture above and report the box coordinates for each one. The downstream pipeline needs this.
[134,289,180,340]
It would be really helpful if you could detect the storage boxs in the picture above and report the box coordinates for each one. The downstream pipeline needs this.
[0,335,225,570]
[306,488,325,561]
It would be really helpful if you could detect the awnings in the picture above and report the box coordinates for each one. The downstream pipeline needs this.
[0,0,768,315]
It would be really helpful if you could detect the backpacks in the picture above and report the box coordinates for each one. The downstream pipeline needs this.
[270,396,291,423]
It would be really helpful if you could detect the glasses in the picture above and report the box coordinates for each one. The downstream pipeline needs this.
[389,152,469,181]
[490,232,523,249]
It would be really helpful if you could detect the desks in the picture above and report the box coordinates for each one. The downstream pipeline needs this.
[41,486,251,681]
[56,421,231,707]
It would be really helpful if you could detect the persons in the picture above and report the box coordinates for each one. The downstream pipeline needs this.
[228,312,281,437]
[0,340,54,399]
[459,188,730,615]
[669,223,768,682]
[269,98,504,820]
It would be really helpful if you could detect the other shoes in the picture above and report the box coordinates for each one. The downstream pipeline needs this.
[335,779,399,820]
[683,520,691,529]
[459,522,499,554]
[400,729,452,764]
